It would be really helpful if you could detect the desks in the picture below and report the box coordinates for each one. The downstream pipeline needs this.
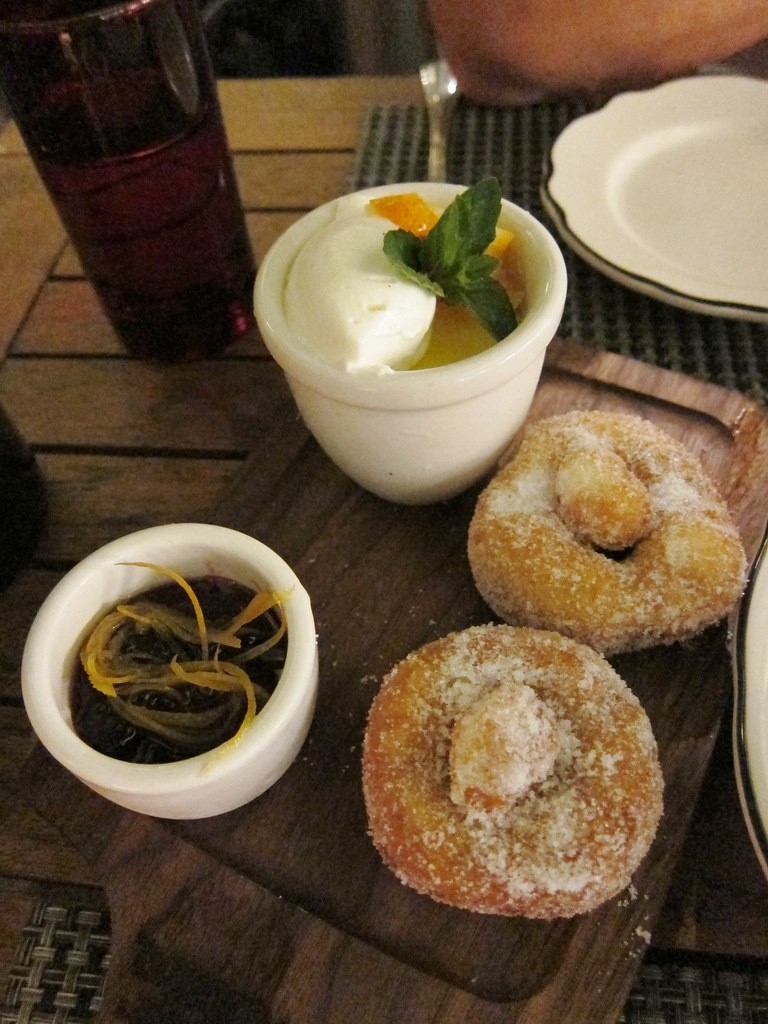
[0,76,768,1024]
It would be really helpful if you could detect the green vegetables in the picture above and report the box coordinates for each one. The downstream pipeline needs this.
[384,175,519,343]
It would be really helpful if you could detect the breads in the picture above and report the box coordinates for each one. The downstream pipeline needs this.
[470,411,749,653]
[364,628,666,914]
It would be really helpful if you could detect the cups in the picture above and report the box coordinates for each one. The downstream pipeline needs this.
[0,408,46,581]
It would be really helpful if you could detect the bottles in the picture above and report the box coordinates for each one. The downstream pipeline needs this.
[0,0,257,355]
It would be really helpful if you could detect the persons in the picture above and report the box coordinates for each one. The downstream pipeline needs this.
[426,0,768,108]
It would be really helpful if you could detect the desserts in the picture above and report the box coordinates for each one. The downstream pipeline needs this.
[283,211,439,373]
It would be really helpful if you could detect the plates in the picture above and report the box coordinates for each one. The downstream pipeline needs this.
[731,520,767,872]
[544,75,767,324]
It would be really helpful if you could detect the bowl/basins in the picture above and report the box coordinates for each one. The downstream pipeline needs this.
[20,523,321,821]
[253,184,564,508]
